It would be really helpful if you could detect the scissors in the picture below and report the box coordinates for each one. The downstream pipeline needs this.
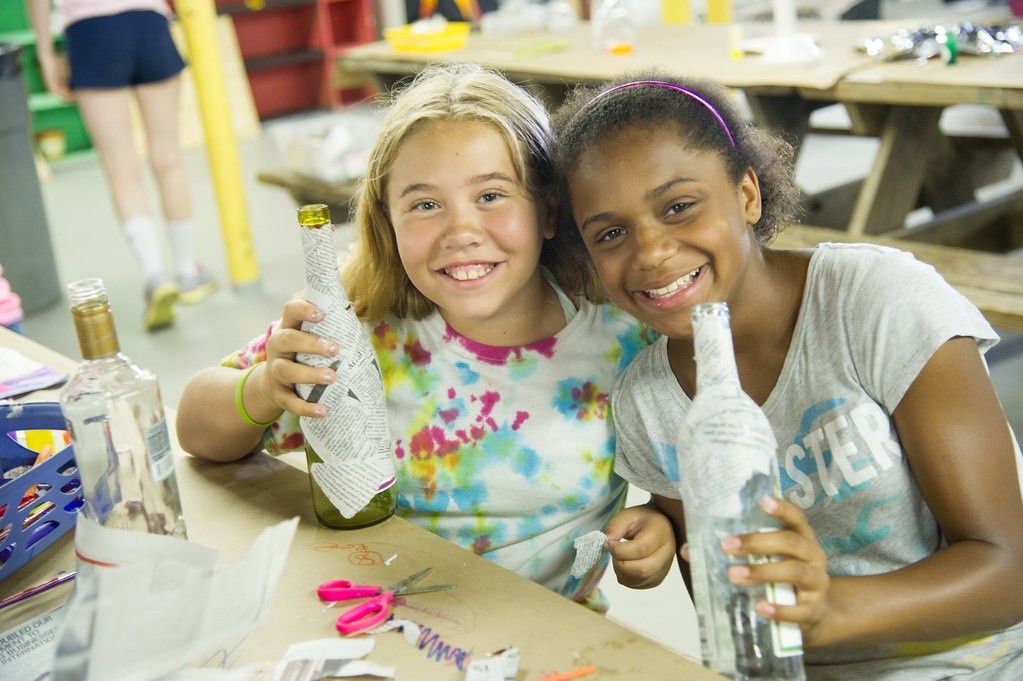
[315,567,455,632]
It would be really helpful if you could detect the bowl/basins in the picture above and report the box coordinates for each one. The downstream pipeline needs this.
[384,21,468,52]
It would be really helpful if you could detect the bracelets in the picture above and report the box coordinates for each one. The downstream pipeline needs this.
[236,361,285,425]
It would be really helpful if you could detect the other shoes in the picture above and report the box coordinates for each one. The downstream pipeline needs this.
[142,277,178,332]
[176,266,217,305]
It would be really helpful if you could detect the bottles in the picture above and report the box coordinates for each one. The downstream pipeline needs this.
[59,278,187,541]
[298,203,396,529]
[676,303,809,681]
[594,0,639,59]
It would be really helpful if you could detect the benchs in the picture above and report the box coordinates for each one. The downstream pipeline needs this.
[742,104,1016,148]
[252,152,1023,334]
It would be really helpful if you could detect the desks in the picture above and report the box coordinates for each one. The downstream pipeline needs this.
[0,327,731,680]
[336,18,1023,234]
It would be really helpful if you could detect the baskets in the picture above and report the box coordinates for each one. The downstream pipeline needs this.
[0,402,87,581]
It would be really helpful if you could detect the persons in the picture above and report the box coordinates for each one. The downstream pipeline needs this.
[177,63,675,612]
[555,75,1023,680]
[27,0,217,331]
